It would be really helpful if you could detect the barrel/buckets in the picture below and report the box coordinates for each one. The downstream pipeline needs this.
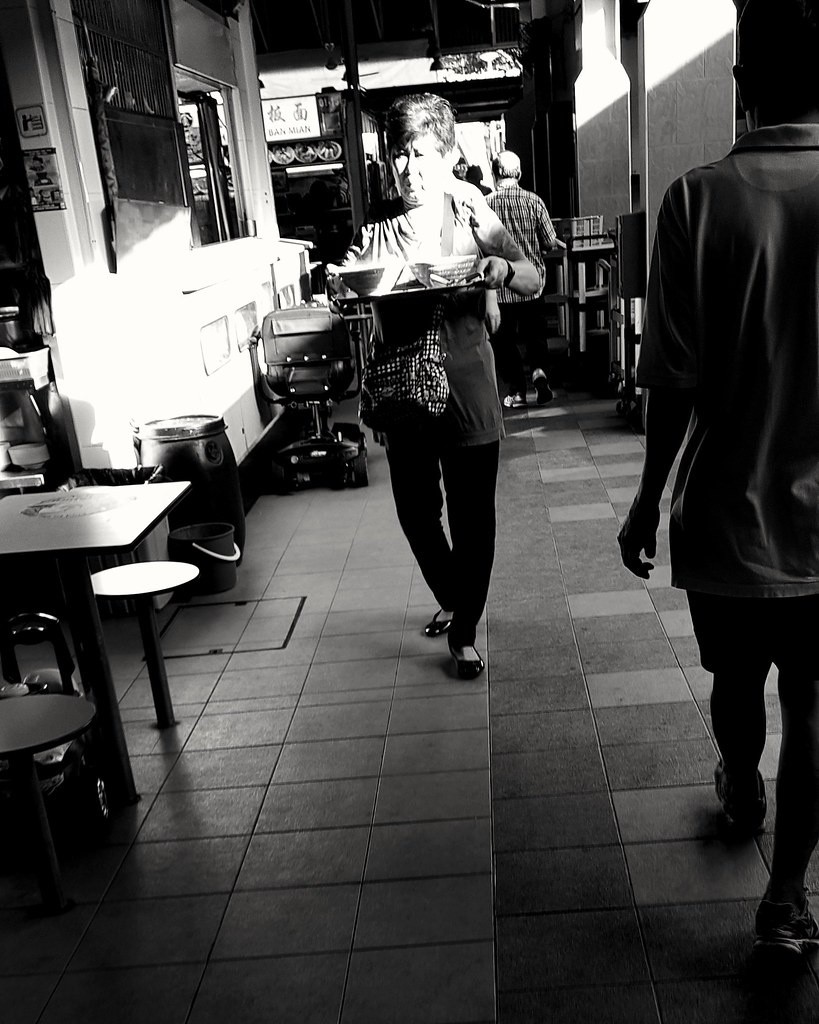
[167,523,241,598]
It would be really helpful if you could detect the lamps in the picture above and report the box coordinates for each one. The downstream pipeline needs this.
[426,31,441,71]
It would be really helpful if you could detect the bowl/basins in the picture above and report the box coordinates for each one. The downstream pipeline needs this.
[338,268,385,297]
[272,145,294,164]
[409,254,477,289]
[294,143,317,163]
[268,150,272,163]
[317,140,342,161]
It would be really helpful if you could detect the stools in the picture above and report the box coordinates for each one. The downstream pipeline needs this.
[0,694,96,920]
[90,561,200,730]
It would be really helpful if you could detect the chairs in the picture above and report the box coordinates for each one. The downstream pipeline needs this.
[250,304,362,407]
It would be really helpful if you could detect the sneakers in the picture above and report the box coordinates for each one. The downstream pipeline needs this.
[714,762,767,830]
[530,367,554,406]
[503,394,530,408]
[752,895,819,953]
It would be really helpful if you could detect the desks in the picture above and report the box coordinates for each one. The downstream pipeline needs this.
[0,480,191,808]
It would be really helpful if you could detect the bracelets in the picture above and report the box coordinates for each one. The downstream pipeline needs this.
[502,260,516,287]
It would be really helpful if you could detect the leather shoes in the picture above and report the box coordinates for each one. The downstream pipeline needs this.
[448,640,484,680]
[425,609,452,636]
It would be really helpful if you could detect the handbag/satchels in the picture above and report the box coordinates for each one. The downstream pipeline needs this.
[357,331,450,436]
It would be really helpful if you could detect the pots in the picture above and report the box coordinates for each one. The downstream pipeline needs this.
[0,312,24,347]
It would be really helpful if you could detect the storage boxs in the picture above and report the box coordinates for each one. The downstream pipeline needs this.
[552,215,604,248]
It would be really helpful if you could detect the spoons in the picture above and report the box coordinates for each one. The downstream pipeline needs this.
[430,272,483,289]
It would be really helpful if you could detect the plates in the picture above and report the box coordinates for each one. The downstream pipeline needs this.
[7,443,49,470]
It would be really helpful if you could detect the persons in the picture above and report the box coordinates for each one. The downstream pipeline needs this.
[480,148,568,408]
[615,0,819,954]
[322,94,548,682]
[466,165,493,197]
